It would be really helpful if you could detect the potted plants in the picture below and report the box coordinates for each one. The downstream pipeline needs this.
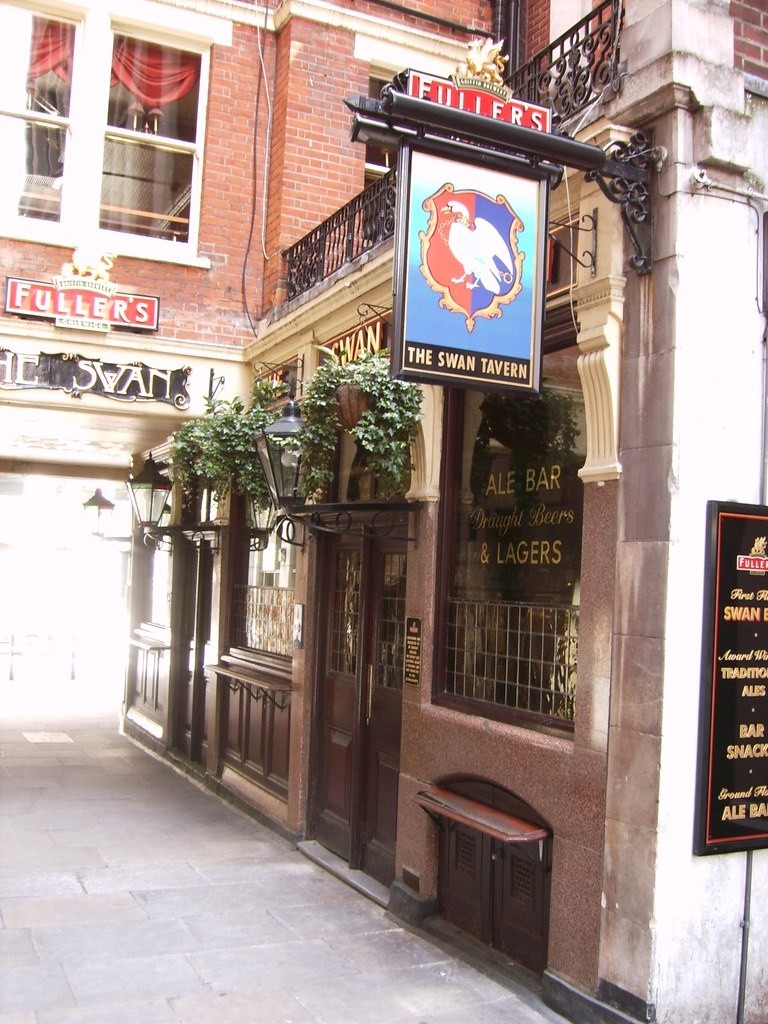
[268,345,426,501]
[481,386,584,521]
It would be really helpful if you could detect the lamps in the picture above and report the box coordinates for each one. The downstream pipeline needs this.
[125,100,165,134]
[81,488,131,558]
[254,354,306,467]
[125,450,220,555]
